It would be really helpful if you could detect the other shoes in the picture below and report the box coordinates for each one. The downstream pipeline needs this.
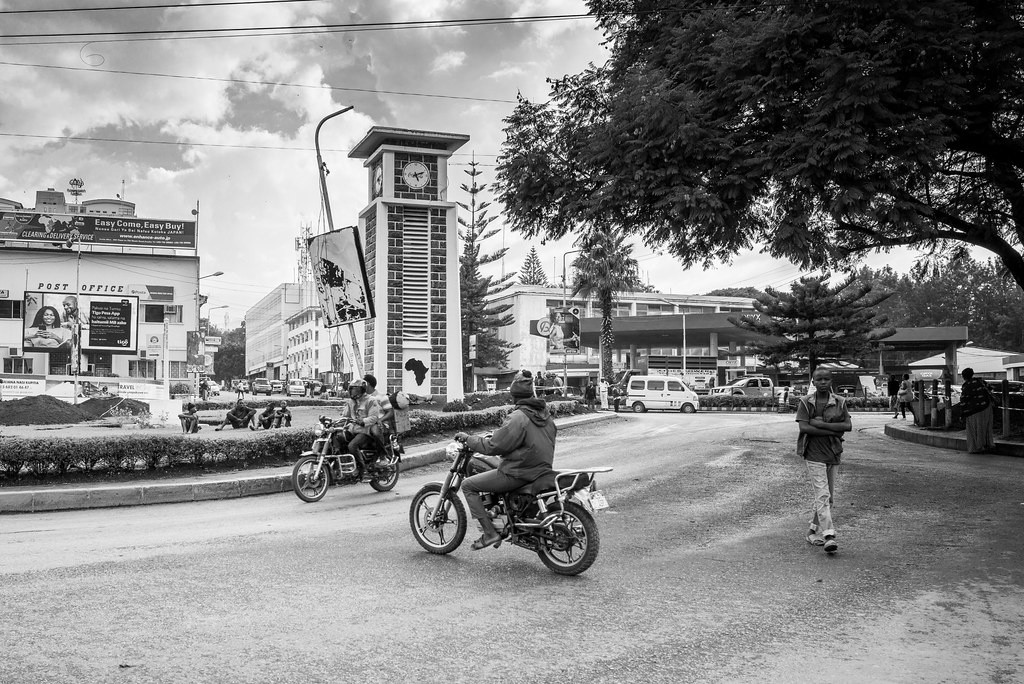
[250,425,256,431]
[894,413,899,419]
[374,459,390,468]
[215,425,223,430]
[359,474,372,483]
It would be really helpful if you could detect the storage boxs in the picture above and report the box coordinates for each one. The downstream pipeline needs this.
[390,407,412,433]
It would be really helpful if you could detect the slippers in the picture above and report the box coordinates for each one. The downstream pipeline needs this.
[824,540,838,554]
[806,534,825,545]
[471,534,502,551]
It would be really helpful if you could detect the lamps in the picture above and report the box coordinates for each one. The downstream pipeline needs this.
[402,160,431,189]
[373,166,382,194]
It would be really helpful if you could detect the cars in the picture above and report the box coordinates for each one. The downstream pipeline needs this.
[303,381,350,397]
[206,381,220,396]
[927,384,963,398]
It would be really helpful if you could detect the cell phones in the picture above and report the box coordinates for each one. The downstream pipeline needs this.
[39,323,47,337]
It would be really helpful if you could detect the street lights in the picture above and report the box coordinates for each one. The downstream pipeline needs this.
[563,244,604,394]
[207,306,229,336]
[198,271,222,332]
[660,298,686,379]
[67,235,80,406]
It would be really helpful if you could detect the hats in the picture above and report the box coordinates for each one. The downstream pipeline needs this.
[188,403,195,410]
[510,371,534,397]
[268,403,275,409]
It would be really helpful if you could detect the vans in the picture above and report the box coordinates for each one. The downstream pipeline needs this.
[626,375,699,414]
[269,379,305,397]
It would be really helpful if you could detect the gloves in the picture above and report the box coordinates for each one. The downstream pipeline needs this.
[356,419,364,426]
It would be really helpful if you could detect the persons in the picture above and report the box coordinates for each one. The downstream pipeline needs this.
[844,388,848,397]
[178,403,198,433]
[535,371,564,397]
[201,380,210,400]
[215,399,258,431]
[78,381,114,397]
[960,367,996,453]
[306,381,315,398]
[237,380,245,399]
[898,374,918,426]
[612,385,621,412]
[586,381,597,410]
[795,367,852,551]
[778,386,789,405]
[319,375,394,481]
[888,375,900,418]
[454,369,557,551]
[24,296,90,349]
[250,401,292,431]
[600,377,609,410]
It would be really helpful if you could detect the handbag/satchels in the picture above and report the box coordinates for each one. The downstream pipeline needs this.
[990,394,1000,412]
[898,380,908,395]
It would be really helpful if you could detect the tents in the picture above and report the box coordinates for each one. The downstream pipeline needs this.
[908,339,1021,379]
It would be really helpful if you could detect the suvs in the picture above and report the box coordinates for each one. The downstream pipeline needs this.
[230,380,250,393]
[252,378,272,396]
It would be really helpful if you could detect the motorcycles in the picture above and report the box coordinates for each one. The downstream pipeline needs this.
[292,414,404,503]
[409,436,614,576]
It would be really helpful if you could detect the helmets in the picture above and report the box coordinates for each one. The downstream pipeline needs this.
[348,379,367,399]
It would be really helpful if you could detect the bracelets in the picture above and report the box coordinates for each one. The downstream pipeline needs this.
[466,436,468,439]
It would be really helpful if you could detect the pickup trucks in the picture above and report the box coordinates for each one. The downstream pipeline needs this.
[707,376,794,397]
